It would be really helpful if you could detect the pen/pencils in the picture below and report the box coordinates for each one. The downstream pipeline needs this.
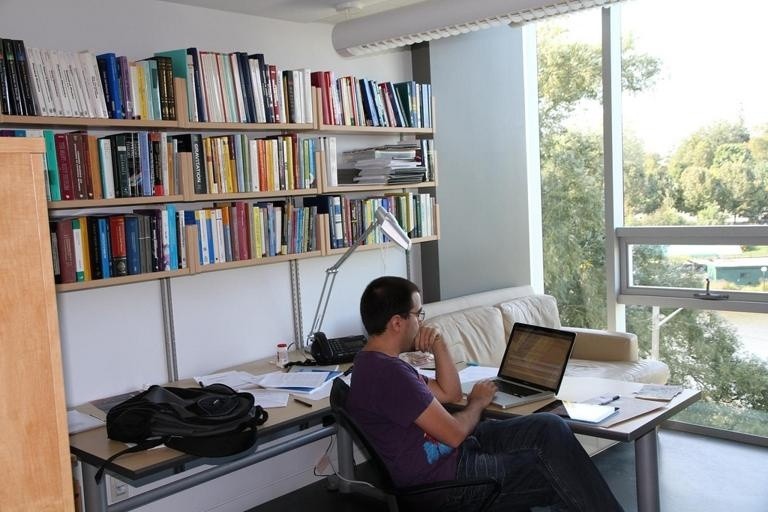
[294,398,312,408]
[599,396,620,405]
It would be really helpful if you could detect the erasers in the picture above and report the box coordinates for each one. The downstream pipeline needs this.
[466,361,480,366]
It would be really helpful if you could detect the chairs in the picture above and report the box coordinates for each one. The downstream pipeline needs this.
[329,372,501,511]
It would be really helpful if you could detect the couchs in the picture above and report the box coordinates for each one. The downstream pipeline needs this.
[390,283,673,460]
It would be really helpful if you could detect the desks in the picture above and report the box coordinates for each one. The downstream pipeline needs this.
[65,332,388,511]
[435,371,701,510]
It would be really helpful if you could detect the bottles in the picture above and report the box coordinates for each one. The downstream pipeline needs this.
[276,343,288,367]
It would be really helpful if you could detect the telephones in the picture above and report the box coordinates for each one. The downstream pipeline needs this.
[312,333,368,366]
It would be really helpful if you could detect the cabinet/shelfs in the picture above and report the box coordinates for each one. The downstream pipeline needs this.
[0,136,77,512]
[0,97,441,294]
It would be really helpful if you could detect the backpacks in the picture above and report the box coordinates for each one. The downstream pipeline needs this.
[106,381,268,457]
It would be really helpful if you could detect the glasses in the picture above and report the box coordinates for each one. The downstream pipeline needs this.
[409,311,425,322]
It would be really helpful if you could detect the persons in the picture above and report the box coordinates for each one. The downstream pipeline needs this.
[341,277,625,511]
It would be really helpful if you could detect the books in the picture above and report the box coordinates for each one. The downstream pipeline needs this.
[287,363,340,374]
[420,359,466,372]
[49,191,435,285]
[194,370,258,392]
[1,128,436,204]
[1,38,435,128]
[76,390,142,422]
[266,365,341,393]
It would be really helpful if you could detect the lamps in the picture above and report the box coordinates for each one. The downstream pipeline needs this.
[329,0,626,59]
[307,204,415,351]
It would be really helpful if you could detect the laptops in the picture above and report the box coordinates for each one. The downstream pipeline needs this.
[460,322,578,410]
